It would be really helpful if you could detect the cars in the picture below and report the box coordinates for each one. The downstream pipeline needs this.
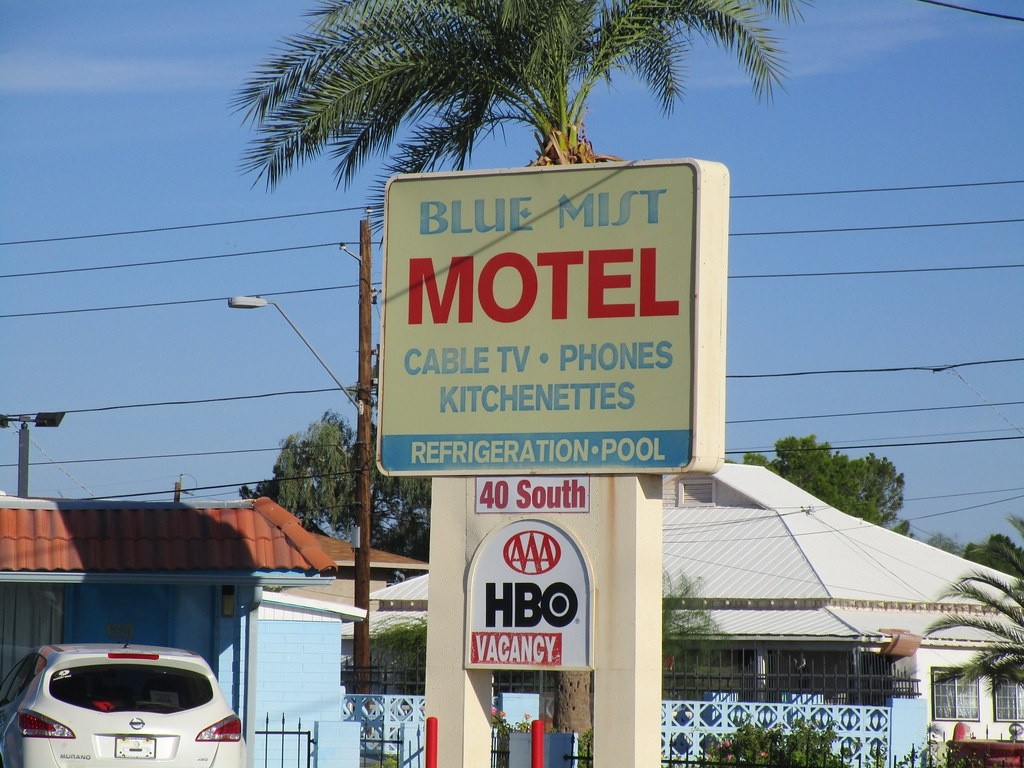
[2,641,248,768]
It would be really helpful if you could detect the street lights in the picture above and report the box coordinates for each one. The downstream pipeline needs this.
[0,411,67,498]
[228,295,373,695]
